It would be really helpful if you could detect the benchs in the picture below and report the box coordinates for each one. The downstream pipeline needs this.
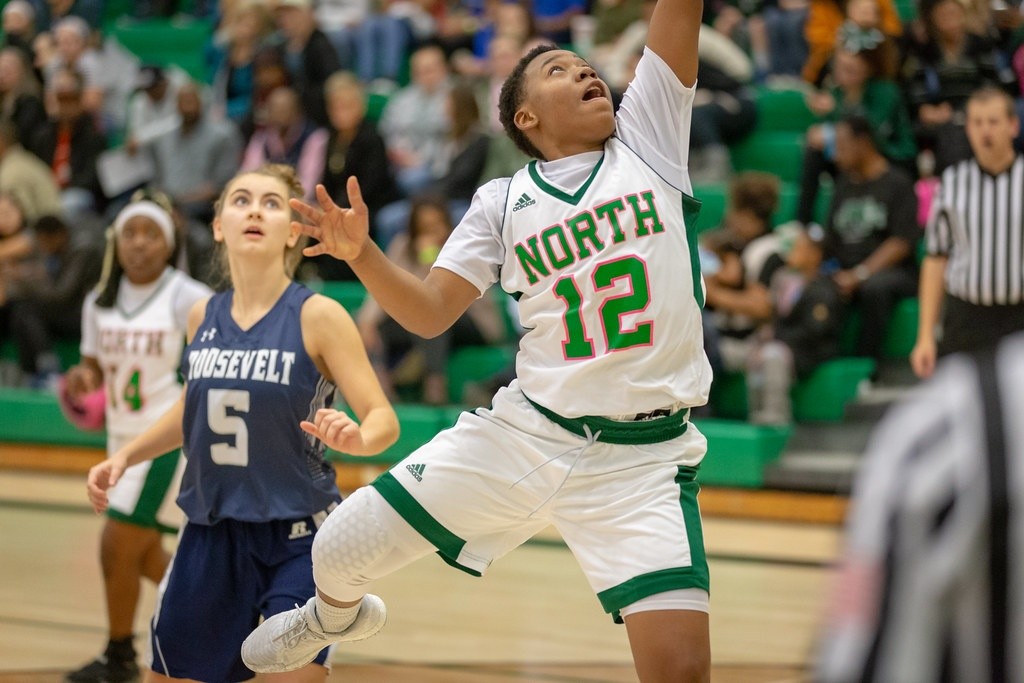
[0,0,921,488]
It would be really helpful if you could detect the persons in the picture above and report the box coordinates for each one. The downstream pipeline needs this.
[60,189,216,683]
[809,329,1023,682]
[240,1,712,683]
[909,84,1023,378]
[0,0,1024,429]
[87,164,402,682]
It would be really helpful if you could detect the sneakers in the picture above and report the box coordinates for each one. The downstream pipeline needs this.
[241,593,386,673]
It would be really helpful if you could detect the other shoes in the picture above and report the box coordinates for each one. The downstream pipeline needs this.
[689,145,734,183]
[67,651,141,683]
